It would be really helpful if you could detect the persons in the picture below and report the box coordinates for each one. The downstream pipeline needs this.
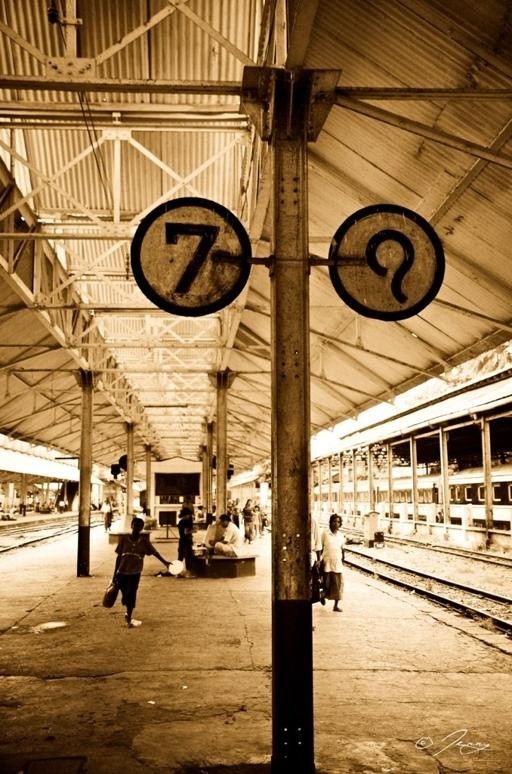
[100,496,112,531]
[227,497,268,543]
[0,497,68,519]
[110,518,174,628]
[319,513,347,613]
[176,506,199,578]
[195,505,205,522]
[210,505,217,517]
[309,514,323,568]
[182,496,194,516]
[209,513,245,558]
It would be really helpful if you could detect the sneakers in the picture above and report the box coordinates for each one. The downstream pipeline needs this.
[320,591,325,605]
[177,574,197,578]
[105,529,111,533]
[244,538,253,544]
[334,607,341,612]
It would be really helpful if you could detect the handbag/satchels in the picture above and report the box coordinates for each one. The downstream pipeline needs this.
[103,581,118,608]
[312,560,320,603]
